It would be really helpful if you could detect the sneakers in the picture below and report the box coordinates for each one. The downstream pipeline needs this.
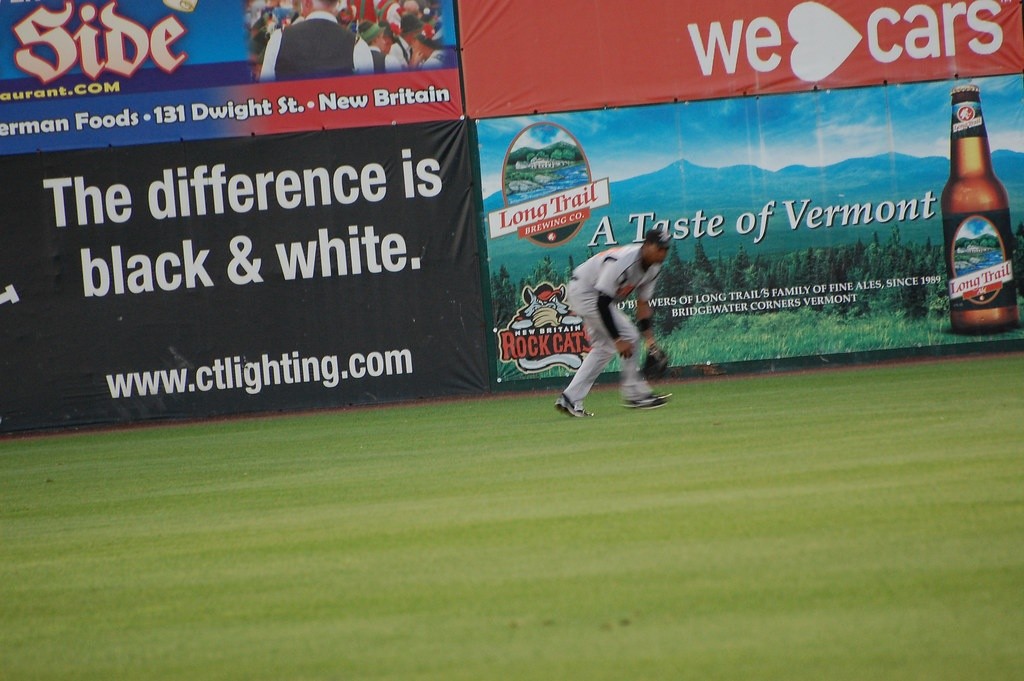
[554,392,595,417]
[623,392,673,409]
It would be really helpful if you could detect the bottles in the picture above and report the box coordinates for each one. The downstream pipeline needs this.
[939,84,1021,335]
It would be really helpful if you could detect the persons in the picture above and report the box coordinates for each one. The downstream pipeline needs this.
[251,0,444,81]
[554,229,671,419]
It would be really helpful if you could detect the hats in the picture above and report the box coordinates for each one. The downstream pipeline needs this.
[401,14,423,34]
[646,229,675,248]
[358,22,380,43]
